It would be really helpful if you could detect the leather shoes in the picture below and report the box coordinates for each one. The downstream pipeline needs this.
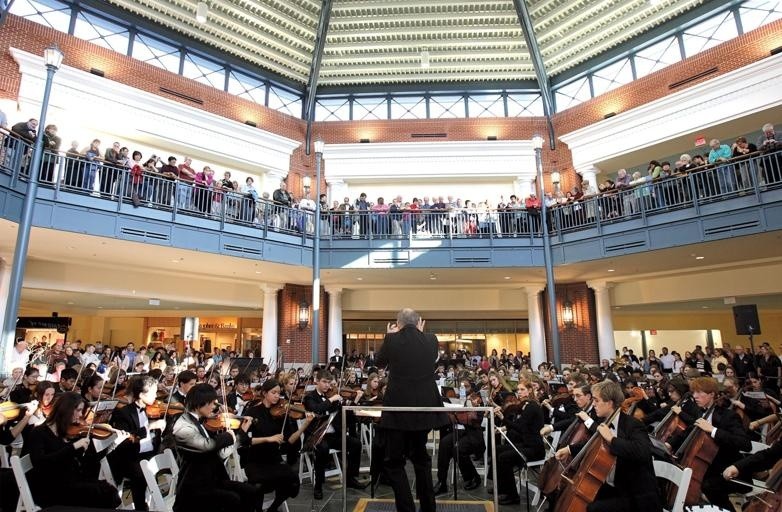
[433,481,448,496]
[489,487,520,504]
[346,477,365,489]
[464,476,482,490]
[314,486,322,499]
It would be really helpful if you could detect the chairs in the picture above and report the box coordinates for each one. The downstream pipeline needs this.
[1,331,780,509]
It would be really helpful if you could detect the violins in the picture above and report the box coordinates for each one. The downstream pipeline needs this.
[155,383,171,399]
[290,389,306,401]
[326,384,366,398]
[454,383,572,416]
[42,398,57,420]
[267,396,323,420]
[66,421,140,444]
[71,385,80,392]
[96,392,130,409]
[241,386,263,401]
[102,379,134,395]
[145,399,185,419]
[29,380,40,394]
[204,411,259,433]
[0,399,41,426]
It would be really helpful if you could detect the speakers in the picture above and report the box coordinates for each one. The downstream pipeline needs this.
[731,304,761,335]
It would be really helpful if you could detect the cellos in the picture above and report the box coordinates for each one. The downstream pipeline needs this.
[537,358,782,511]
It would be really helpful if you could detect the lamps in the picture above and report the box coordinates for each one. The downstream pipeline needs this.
[360,133,368,142]
[245,119,258,125]
[195,0,209,27]
[90,66,105,78]
[551,168,561,192]
[300,293,312,330]
[769,46,780,55]
[488,133,498,140]
[563,298,578,332]
[603,110,615,119]
[302,172,312,190]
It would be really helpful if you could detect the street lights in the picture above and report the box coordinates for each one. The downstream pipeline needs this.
[0,38,66,379]
[532,133,568,370]
[302,131,327,371]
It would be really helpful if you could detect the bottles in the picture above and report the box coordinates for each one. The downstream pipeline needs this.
[459,382,466,401]
